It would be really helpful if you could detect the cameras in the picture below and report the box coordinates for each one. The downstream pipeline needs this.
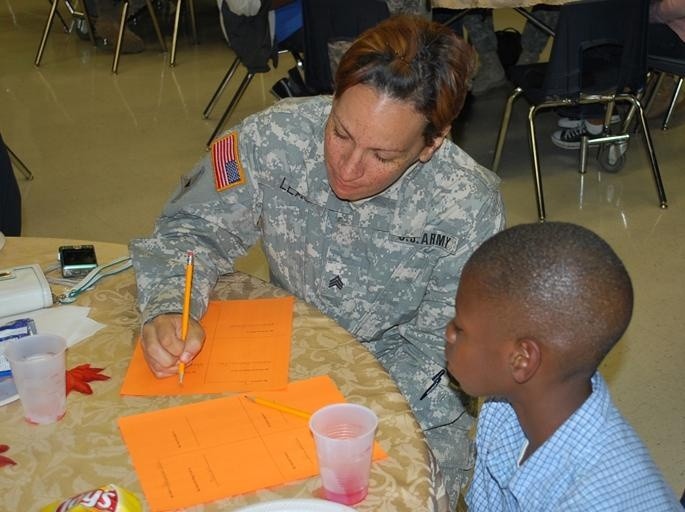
[58,245,98,278]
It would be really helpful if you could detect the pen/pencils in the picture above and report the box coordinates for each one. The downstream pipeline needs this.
[244,395,312,420]
[176,250,193,383]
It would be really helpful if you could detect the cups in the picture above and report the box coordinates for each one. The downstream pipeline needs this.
[308,402,379,506]
[4,334,66,424]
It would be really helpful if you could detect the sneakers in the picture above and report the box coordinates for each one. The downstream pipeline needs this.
[93,12,145,54]
[557,107,621,129]
[648,83,683,117]
[77,14,90,40]
[551,121,612,150]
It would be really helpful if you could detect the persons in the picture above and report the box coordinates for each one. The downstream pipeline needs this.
[130,11,506,512]
[78,1,142,54]
[444,219,685,512]
[227,1,685,173]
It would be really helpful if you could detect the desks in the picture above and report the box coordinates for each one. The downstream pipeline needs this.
[0,235,432,512]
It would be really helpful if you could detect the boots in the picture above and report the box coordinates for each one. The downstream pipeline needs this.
[469,53,508,92]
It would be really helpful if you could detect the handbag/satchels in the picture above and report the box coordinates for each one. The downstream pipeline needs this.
[1,255,133,318]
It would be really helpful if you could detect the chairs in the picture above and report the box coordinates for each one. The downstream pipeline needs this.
[303,1,393,100]
[108,2,197,74]
[203,0,311,152]
[635,24,685,134]
[36,2,97,65]
[491,2,667,221]
